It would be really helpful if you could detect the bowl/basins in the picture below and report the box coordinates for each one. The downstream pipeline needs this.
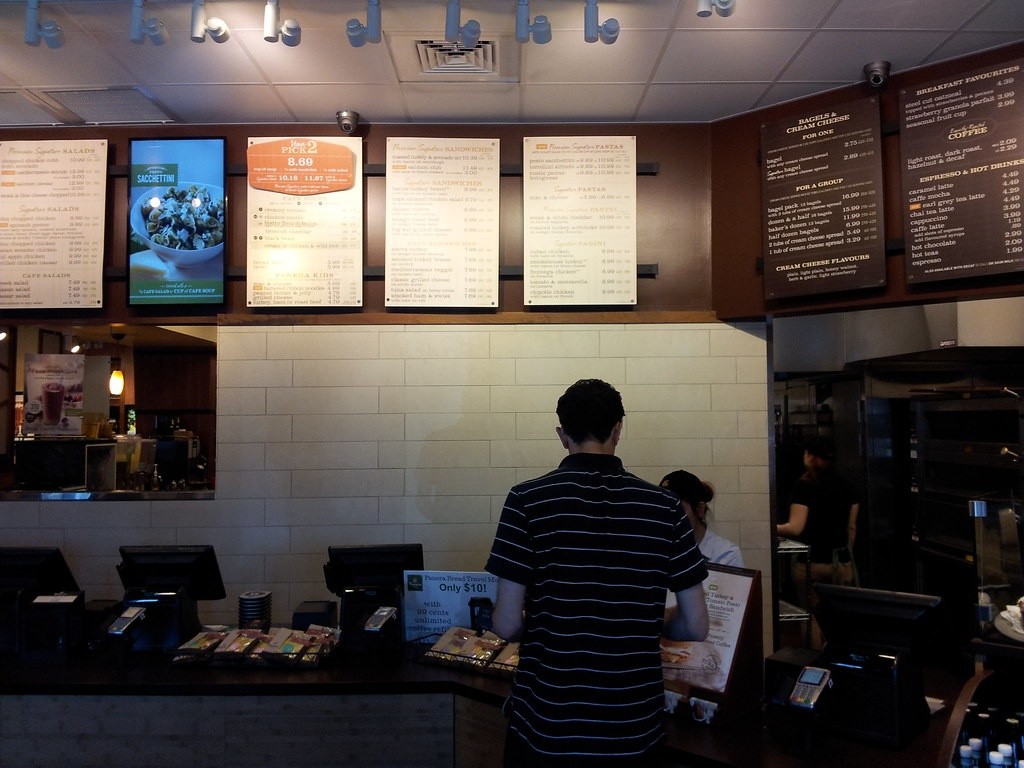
[130,182,223,269]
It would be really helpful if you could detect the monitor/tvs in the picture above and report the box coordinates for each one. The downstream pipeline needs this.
[118,546,227,601]
[0,546,81,595]
[328,544,425,595]
[815,585,948,673]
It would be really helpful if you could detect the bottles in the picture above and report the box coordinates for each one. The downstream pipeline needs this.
[134,464,185,492]
[170,417,180,436]
[959,700,1024,768]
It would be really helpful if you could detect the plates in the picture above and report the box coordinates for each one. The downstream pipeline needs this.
[994,606,1024,643]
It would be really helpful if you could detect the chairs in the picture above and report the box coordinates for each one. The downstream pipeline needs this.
[59,439,157,494]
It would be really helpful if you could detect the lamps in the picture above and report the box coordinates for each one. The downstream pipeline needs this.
[264,0,301,46]
[583,0,620,45]
[345,0,381,48]
[26,0,64,49]
[446,0,481,48]
[515,0,552,44]
[109,333,126,396]
[65,335,80,354]
[129,0,170,46]
[191,0,231,43]
[696,0,736,17]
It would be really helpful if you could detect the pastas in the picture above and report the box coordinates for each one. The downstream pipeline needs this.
[142,186,225,250]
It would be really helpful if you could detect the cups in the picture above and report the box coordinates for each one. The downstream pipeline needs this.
[40,382,65,426]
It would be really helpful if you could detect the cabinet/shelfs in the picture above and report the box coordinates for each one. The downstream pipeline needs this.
[775,538,812,650]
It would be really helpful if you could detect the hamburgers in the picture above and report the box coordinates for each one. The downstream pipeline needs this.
[660,638,693,663]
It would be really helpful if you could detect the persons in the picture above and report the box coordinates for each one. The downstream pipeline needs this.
[776,438,861,652]
[483,380,710,768]
[654,470,747,572]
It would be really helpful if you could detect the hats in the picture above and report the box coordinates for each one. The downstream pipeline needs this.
[659,469,706,495]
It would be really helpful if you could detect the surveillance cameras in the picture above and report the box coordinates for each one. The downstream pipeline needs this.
[337,111,359,133]
